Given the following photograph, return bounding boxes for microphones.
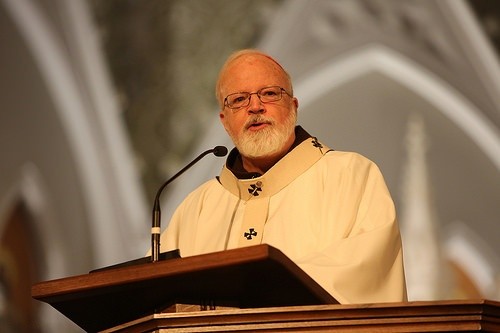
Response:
[152,146,228,262]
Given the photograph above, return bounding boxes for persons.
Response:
[146,50,409,302]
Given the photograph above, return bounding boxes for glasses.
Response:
[223,85,293,109]
